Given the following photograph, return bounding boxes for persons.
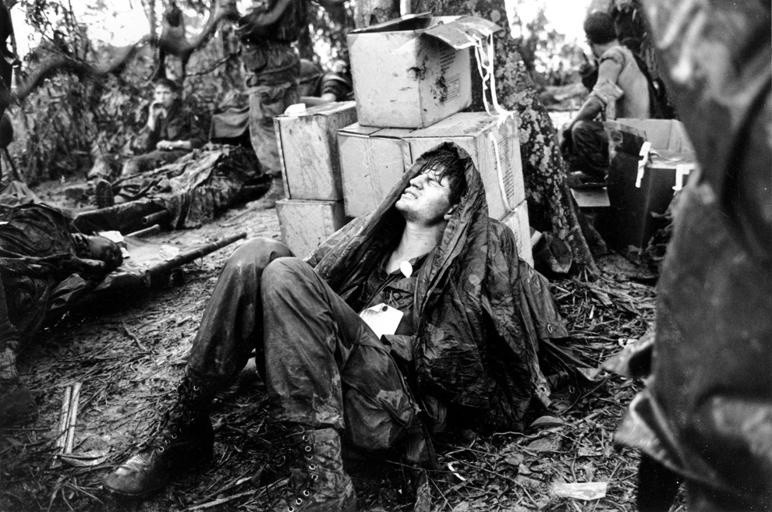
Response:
[89,77,203,211]
[563,12,650,191]
[622,2,771,507]
[297,59,354,106]
[234,0,311,210]
[574,40,599,94]
[101,142,538,512]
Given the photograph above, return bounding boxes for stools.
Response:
[570,186,611,255]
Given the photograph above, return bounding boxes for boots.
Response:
[245,176,286,210]
[275,425,356,511]
[100,367,214,495]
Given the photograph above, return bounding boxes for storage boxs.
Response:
[278,102,358,201]
[408,110,528,222]
[504,200,535,269]
[345,14,503,125]
[278,196,342,261]
[337,124,409,222]
[607,117,697,252]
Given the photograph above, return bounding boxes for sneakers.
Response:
[565,170,607,191]
[62,175,116,209]
